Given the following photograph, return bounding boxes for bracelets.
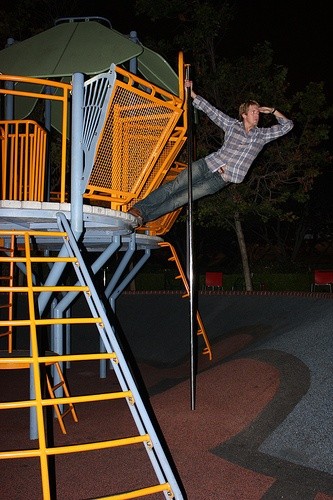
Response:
[271,108,276,114]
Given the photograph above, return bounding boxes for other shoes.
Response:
[129,208,144,226]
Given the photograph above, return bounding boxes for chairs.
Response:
[203,270,224,292]
[309,266,333,293]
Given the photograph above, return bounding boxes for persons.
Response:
[130,80,294,226]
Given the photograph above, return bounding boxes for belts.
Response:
[217,168,224,176]
